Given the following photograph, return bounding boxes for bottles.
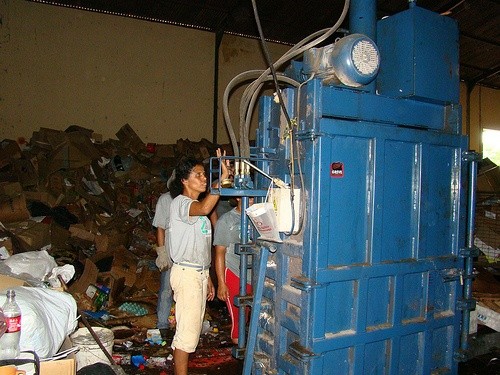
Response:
[0,291,22,358]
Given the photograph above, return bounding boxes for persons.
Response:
[151,165,255,346]
[169,148,230,375]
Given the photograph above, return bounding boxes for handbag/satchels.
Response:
[246,179,282,244]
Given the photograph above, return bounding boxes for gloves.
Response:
[155,246,170,272]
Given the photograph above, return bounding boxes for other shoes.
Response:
[161,329,172,338]
[232,343,240,358]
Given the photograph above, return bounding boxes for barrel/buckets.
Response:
[70,327,114,371]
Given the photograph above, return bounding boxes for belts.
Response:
[175,263,211,272]
